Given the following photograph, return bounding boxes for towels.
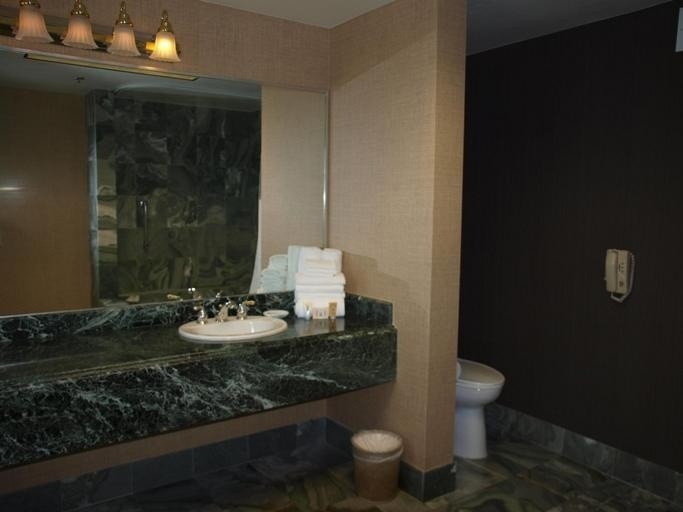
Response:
[256,245,298,295]
[293,247,347,321]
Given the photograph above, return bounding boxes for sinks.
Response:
[177,314,289,344]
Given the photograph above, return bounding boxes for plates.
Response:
[263,309,290,318]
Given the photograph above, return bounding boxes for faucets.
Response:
[188,287,203,298]
[215,299,240,322]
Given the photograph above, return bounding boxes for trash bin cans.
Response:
[350,428,406,501]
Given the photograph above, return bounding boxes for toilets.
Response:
[451,356,505,462]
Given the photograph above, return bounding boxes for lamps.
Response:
[15,0,183,63]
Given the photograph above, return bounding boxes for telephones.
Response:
[604,247,636,303]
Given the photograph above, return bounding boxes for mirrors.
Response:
[0,45,330,320]
